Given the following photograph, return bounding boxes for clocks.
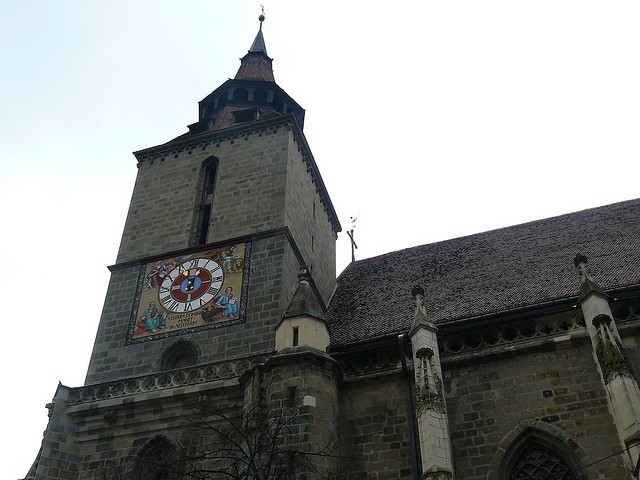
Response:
[157,257,225,314]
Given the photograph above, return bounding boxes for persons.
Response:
[220,245,242,274]
[217,287,238,319]
[141,301,160,332]
[147,260,166,287]
